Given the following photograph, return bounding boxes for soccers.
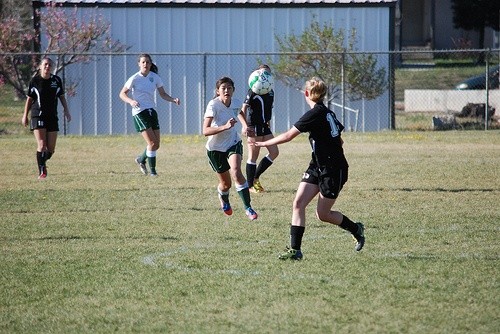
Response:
[247,68,274,95]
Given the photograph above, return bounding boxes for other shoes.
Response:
[37,165,48,177]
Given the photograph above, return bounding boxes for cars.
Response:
[454,65,500,90]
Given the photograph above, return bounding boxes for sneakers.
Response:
[217,196,233,216]
[277,247,302,260]
[250,179,264,195]
[352,222,365,250]
[149,169,158,176]
[244,207,258,219]
[136,155,146,173]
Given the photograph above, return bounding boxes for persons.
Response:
[119,54,181,178]
[201,76,258,221]
[237,63,279,196]
[22,57,73,180]
[247,77,366,262]
